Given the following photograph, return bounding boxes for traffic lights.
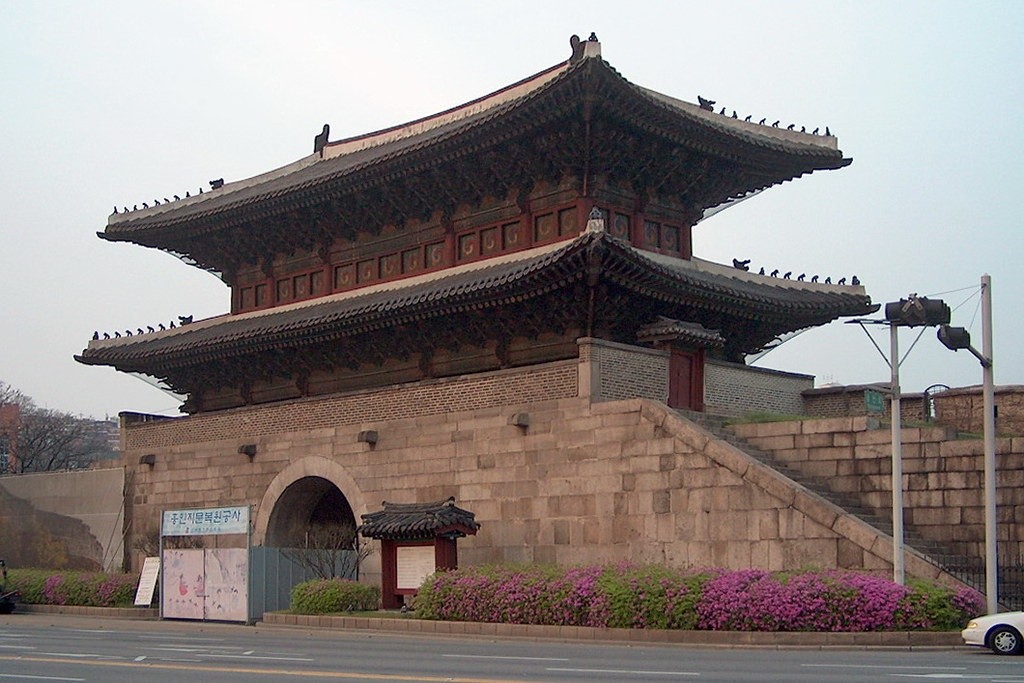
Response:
[917,296,952,328]
[938,327,972,354]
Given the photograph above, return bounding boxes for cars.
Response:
[961,610,1024,655]
